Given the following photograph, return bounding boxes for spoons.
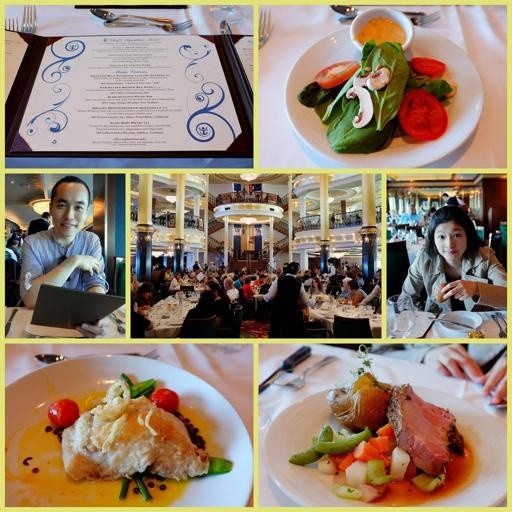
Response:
[111,313,126,335]
[492,314,507,339]
[34,352,68,365]
[89,8,175,23]
[329,4,426,18]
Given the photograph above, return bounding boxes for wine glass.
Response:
[407,234,415,249]
[397,229,407,242]
[422,227,429,243]
[388,294,416,338]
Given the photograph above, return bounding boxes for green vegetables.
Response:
[327,96,398,153]
[426,79,453,96]
[408,77,429,89]
[133,468,164,500]
[297,80,336,107]
[361,39,376,71]
[364,41,410,132]
[118,476,131,500]
[321,65,363,125]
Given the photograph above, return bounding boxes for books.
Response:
[28,283,124,326]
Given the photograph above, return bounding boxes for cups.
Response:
[176,292,183,306]
[255,290,261,296]
[160,302,169,317]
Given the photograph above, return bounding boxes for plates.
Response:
[391,310,437,339]
[4,353,254,508]
[170,319,183,326]
[254,295,266,298]
[262,386,508,509]
[439,310,484,332]
[283,22,485,171]
[25,312,97,339]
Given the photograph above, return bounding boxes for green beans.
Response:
[130,378,156,399]
[313,429,371,455]
[289,425,333,466]
[138,386,154,398]
[187,456,233,477]
[120,373,133,387]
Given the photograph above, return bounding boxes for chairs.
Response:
[215,305,241,338]
[387,240,410,303]
[299,310,333,338]
[187,315,216,338]
[333,315,373,338]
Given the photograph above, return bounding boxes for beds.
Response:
[301,294,382,338]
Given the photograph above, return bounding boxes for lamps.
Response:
[213,202,284,225]
[29,199,51,216]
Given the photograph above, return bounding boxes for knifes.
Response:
[5,308,18,338]
[258,345,312,394]
[428,317,474,331]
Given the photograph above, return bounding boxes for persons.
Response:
[203,282,232,337]
[235,268,273,316]
[179,290,217,339]
[19,177,109,336]
[222,277,239,313]
[182,262,228,293]
[257,263,311,339]
[130,266,186,340]
[401,203,425,230]
[375,342,509,408]
[27,212,50,234]
[303,258,344,296]
[398,207,507,312]
[436,192,473,214]
[425,207,436,223]
[341,260,383,315]
[4,228,29,250]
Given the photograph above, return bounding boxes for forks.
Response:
[257,6,271,48]
[103,19,193,32]
[19,3,38,35]
[338,10,442,26]
[4,16,19,33]
[271,354,338,391]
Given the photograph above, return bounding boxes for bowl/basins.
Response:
[349,6,415,53]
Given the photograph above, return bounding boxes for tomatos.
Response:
[149,388,180,413]
[400,90,448,140]
[315,60,360,89]
[409,55,447,83]
[48,397,79,427]
